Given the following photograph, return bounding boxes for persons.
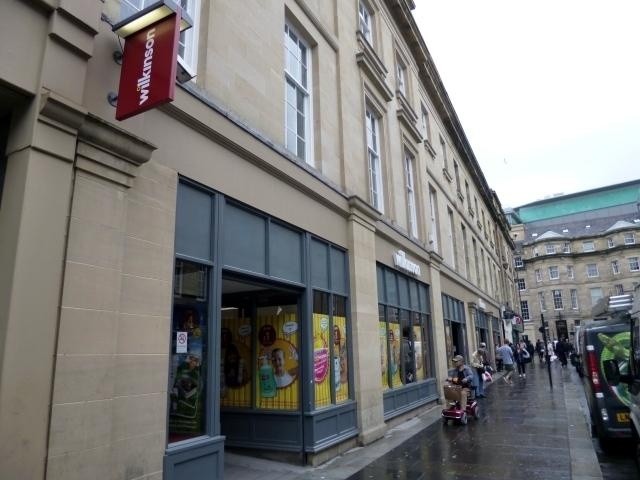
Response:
[271,349,293,389]
[175,355,203,418]
[333,324,343,355]
[513,343,526,379]
[224,343,245,389]
[390,329,397,348]
[451,354,474,413]
[479,343,488,399]
[494,337,570,372]
[496,339,517,386]
[470,347,484,399]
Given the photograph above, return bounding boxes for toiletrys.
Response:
[257,355,277,398]
[334,354,341,392]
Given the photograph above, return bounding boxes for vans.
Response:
[573,282,639,460]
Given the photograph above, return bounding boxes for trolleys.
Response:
[437,375,482,425]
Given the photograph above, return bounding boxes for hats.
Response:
[452,355,464,362]
